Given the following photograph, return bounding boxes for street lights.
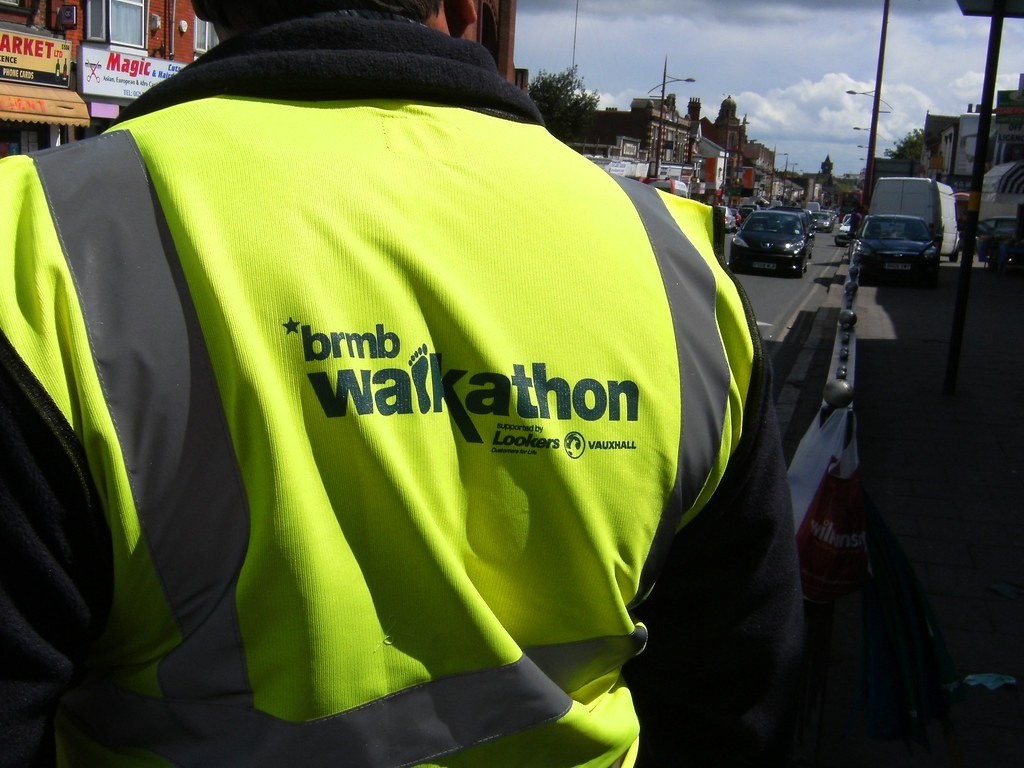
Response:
[846,89,895,207]
[645,54,697,177]
[775,151,805,209]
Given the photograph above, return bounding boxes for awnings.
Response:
[981,160,1024,205]
[0,82,90,128]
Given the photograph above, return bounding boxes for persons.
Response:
[851,208,862,233]
[867,224,883,238]
[0,0,806,768]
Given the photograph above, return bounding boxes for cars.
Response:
[729,207,741,225]
[847,213,944,291]
[725,208,814,278]
[804,199,863,248]
[737,204,759,220]
[717,206,737,234]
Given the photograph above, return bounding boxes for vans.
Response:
[867,176,965,263]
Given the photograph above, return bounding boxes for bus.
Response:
[838,190,864,226]
